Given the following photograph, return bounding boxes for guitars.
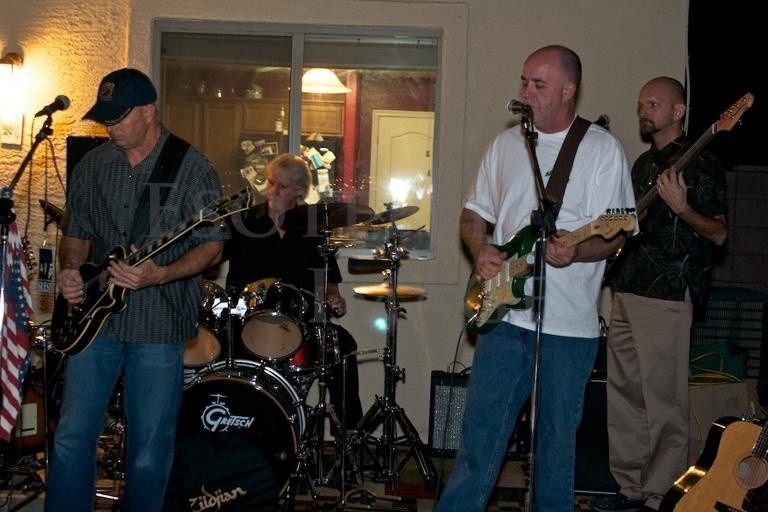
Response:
[604,91,757,274]
[462,208,636,332]
[51,186,258,356]
[658,401,766,511]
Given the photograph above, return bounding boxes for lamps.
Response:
[302,69,352,95]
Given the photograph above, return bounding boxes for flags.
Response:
[0,200,36,444]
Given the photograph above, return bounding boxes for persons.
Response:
[603,76,729,512]
[216,151,375,479]
[433,44,641,512]
[1,187,12,199]
[41,67,227,511]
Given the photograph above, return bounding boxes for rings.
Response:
[657,182,662,188]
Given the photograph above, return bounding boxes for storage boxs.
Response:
[689,378,757,463]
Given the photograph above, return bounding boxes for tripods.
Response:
[302,262,437,511]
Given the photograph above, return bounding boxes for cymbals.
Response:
[38,198,65,228]
[352,283,429,302]
[364,207,420,226]
[278,203,375,231]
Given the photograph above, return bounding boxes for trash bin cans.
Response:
[689,342,750,381]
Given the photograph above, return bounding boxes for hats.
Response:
[79,69,158,126]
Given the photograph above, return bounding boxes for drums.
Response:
[238,280,310,361]
[169,359,307,509]
[183,282,229,366]
[275,321,328,379]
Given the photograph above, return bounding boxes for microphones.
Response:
[506,97,538,116]
[34,96,70,117]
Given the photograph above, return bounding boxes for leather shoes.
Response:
[591,489,640,512]
